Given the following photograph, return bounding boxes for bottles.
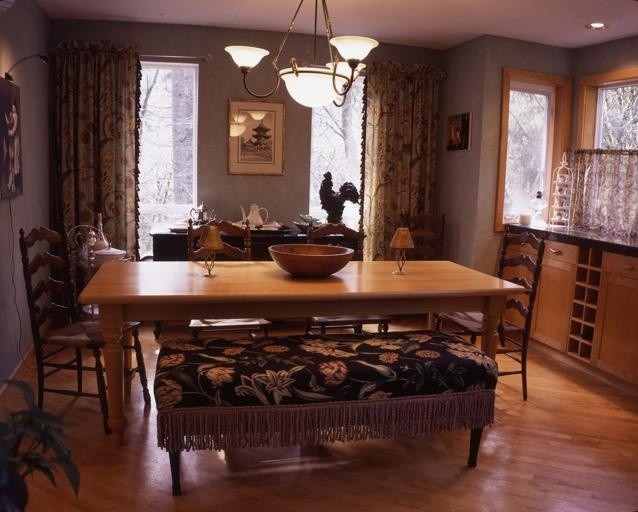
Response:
[94,214,109,250]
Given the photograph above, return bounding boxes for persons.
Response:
[5,101,21,193]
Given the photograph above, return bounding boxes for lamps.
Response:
[222,0,383,112]
[2,54,49,80]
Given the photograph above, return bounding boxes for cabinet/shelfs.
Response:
[506,230,637,387]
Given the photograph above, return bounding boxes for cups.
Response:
[519,208,532,226]
[554,173,572,220]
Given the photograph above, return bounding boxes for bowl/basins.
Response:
[267,243,354,279]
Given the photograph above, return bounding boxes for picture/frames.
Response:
[226,98,286,177]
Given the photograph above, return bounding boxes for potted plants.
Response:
[1,376,83,512]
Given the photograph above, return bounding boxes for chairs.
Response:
[188,218,253,263]
[399,211,547,407]
[17,223,152,438]
[308,219,364,263]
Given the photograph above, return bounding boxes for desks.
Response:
[76,258,527,448]
[150,223,365,260]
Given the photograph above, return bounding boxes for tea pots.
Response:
[190,200,216,225]
[239,202,269,227]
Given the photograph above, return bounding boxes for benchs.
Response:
[151,329,499,497]
